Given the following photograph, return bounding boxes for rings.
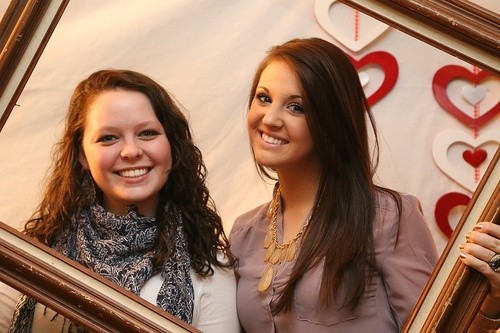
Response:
[490,254,499,272]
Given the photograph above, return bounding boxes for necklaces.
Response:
[258,181,320,292]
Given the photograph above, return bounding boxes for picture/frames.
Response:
[0,0,500,333]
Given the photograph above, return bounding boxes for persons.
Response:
[225,38,500,333]
[18,69,241,333]
